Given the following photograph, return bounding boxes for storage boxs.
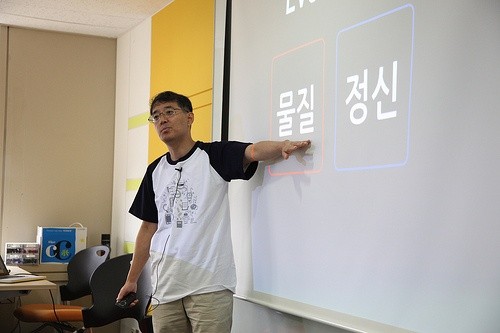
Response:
[37,226,87,264]
[4,242,40,266]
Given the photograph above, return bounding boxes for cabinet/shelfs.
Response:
[0,264,120,333]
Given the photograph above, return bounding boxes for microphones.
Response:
[175,167,183,172]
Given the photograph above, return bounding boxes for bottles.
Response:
[101,234,110,260]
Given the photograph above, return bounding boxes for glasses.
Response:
[148,108,189,122]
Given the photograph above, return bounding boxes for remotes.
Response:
[116,292,136,309]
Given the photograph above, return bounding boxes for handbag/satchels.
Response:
[36,222,87,265]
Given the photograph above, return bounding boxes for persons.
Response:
[116,91,311,333]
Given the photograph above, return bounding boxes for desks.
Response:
[0,266,58,290]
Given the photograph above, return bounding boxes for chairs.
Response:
[13,246,154,333]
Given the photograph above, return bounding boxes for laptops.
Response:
[0,256,47,283]
